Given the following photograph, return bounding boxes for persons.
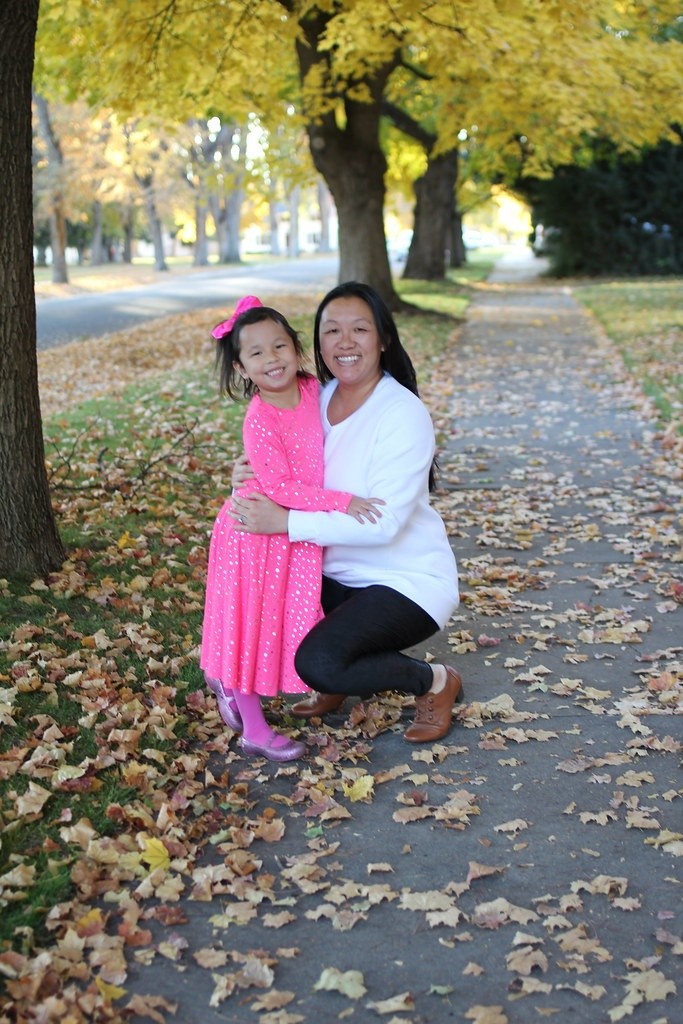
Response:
[227,279,467,744]
[205,302,382,763]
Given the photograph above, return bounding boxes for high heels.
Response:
[291,692,375,717]
[404,663,465,743]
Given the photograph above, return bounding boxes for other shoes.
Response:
[242,733,306,762]
[203,672,243,733]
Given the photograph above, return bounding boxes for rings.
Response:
[239,516,244,524]
